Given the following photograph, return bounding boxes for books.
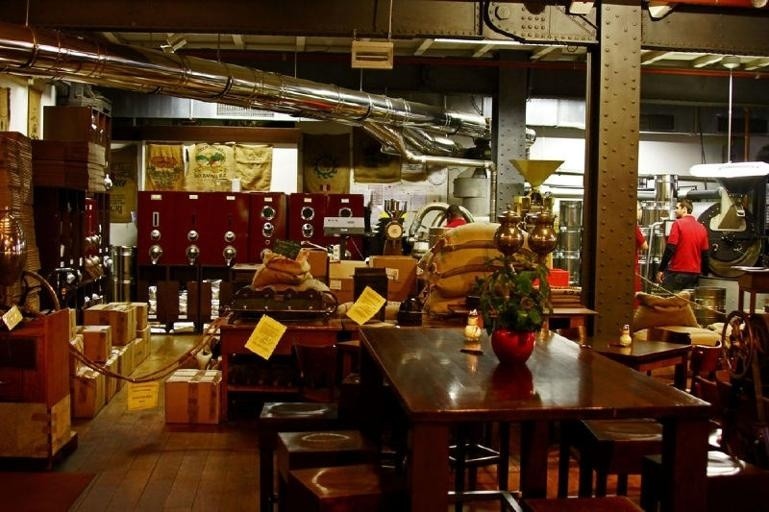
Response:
[163,366,223,428]
[69,298,150,419]
[300,246,419,305]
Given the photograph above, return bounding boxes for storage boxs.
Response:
[300,246,419,305]
[163,366,223,428]
[69,298,150,419]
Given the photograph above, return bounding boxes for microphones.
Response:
[640,449,768,512]
[258,397,401,511]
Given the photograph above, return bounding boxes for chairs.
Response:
[686,339,725,393]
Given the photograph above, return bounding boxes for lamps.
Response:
[686,339,725,393]
[690,67,768,179]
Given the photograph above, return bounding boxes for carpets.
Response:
[1,467,96,511]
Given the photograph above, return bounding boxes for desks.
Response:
[356,326,716,512]
[218,308,344,423]
[737,269,769,316]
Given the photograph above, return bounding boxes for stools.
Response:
[258,397,401,511]
[640,449,768,512]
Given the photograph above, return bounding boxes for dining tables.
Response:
[469,254,553,366]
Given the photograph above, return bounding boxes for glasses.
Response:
[690,67,768,179]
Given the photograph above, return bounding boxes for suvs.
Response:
[696,284,725,325]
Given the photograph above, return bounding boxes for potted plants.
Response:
[469,254,553,366]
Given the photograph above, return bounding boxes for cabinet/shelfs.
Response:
[535,312,552,345]
[60,258,85,286]
[464,313,481,343]
[96,221,111,272]
[618,325,632,348]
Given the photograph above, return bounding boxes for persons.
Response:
[445,205,468,227]
[655,198,710,292]
[633,200,648,311]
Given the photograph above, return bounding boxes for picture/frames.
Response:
[737,269,769,316]
[218,308,344,423]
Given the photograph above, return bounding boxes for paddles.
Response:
[1,467,96,511]
